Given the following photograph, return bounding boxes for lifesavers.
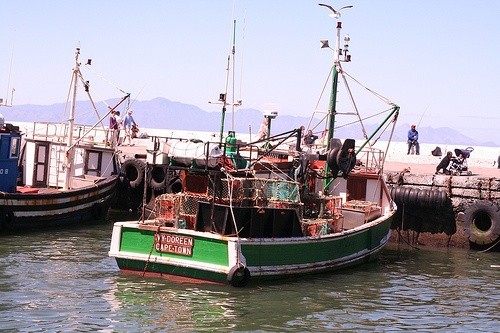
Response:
[145,162,173,192]
[165,175,184,194]
[119,159,146,191]
[461,199,500,245]
[227,265,251,287]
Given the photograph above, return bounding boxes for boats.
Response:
[0,40,120,222]
[108,22,398,288]
[168,56,293,168]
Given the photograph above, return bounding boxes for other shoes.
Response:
[407,152,409,154]
[416,153,419,155]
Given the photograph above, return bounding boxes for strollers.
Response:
[446,147,474,177]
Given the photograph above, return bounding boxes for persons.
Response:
[435,151,452,175]
[110,110,138,147]
[304,130,318,147]
[457,154,463,162]
[407,125,420,155]
[259,118,273,141]
[163,206,187,229]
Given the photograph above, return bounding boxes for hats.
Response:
[411,125,416,128]
[128,109,133,114]
